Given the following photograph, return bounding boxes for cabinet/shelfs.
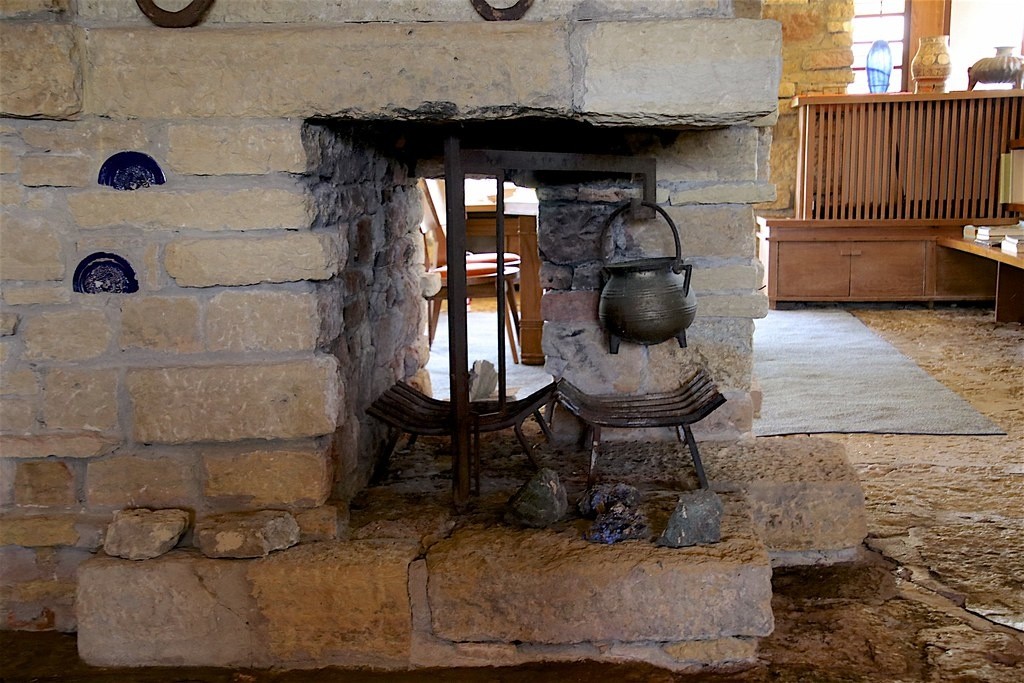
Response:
[755,87,1024,303]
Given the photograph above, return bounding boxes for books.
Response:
[1000,234,1024,255]
[974,223,1024,246]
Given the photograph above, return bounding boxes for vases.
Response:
[910,35,951,92]
[867,39,892,92]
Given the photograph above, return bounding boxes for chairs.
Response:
[417,177,521,364]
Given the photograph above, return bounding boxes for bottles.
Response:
[866,38,893,94]
[911,35,951,94]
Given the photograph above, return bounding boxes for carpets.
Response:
[426,309,1006,435]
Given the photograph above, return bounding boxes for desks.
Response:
[465,193,546,366]
[935,237,1024,323]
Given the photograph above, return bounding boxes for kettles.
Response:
[596,199,697,355]
